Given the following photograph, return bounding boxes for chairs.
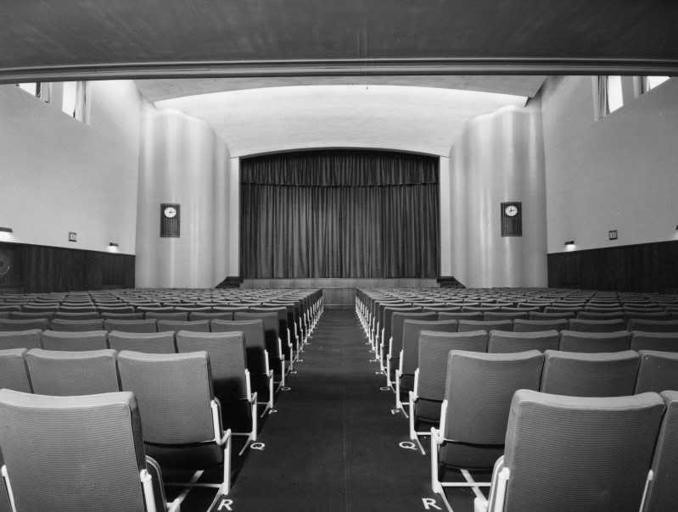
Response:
[407,327,488,443]
[429,348,544,497]
[212,318,277,412]
[1,388,174,512]
[472,388,666,512]
[353,286,678,414]
[176,329,259,456]
[118,349,235,498]
[2,288,325,390]
[25,348,122,398]
[641,388,678,511]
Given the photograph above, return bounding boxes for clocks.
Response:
[160,202,181,237]
[500,201,522,237]
